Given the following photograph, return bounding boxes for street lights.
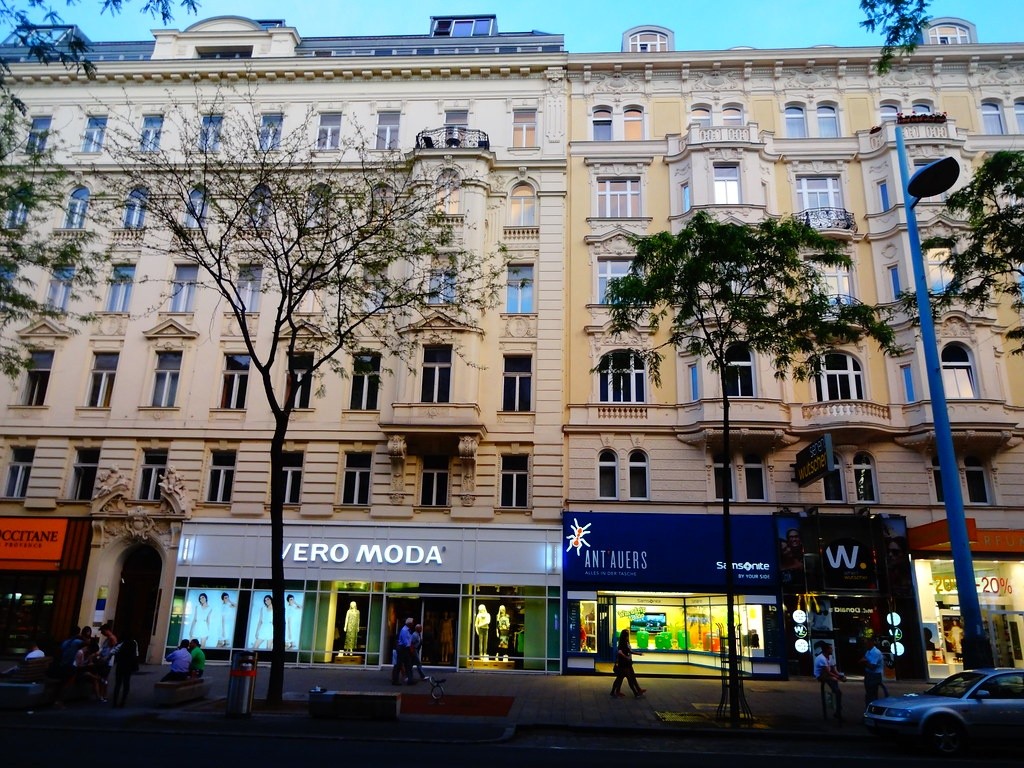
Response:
[894,124,1005,689]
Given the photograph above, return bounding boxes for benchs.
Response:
[310,690,402,719]
[0,683,43,710]
[154,676,214,705]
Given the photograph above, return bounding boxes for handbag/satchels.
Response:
[614,665,621,676]
[66,647,78,664]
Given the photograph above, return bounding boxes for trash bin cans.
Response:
[225,649,258,716]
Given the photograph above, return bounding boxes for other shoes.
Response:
[617,692,625,696]
[392,681,402,686]
[833,713,845,723]
[96,697,108,704]
[639,689,646,695]
[119,703,123,708]
[635,693,639,697]
[112,704,117,708]
[407,679,417,685]
[610,692,615,696]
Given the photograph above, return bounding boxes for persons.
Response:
[858,638,888,701]
[424,612,453,663]
[475,604,491,660]
[814,642,847,721]
[216,592,236,649]
[188,639,205,678]
[951,620,964,663]
[284,594,302,651]
[923,628,935,650]
[889,535,912,586]
[779,528,806,594]
[24,624,140,710]
[160,639,192,683]
[391,618,429,685]
[188,593,212,647]
[610,629,646,699]
[344,601,360,656]
[496,605,510,663]
[253,594,274,650]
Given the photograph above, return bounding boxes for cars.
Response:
[862,666,1024,757]
